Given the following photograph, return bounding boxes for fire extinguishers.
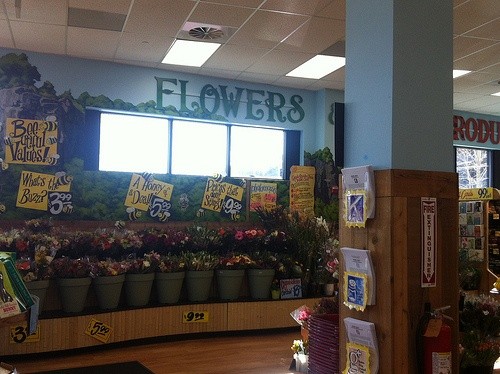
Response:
[423,305,455,374]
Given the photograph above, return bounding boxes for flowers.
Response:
[291,339,308,355]
[459,294,500,374]
[0,205,340,284]
[290,292,339,328]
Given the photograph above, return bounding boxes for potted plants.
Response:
[458,248,482,291]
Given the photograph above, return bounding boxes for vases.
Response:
[324,284,334,295]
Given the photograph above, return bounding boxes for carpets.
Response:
[26,360,154,374]
